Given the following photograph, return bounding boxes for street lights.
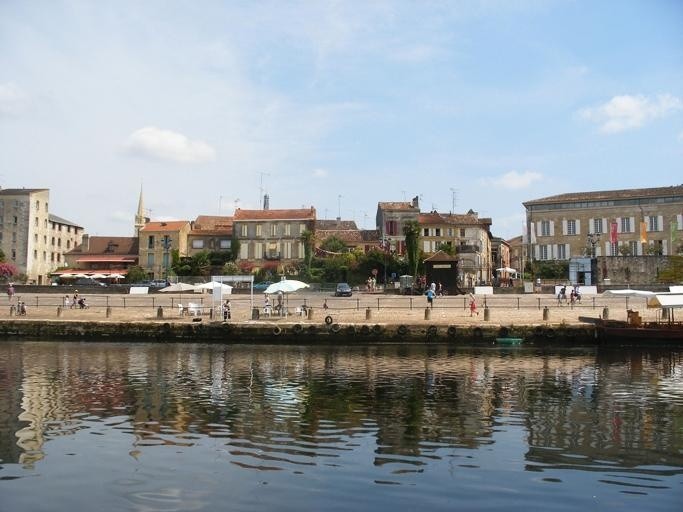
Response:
[380,238,391,284]
[586,232,601,258]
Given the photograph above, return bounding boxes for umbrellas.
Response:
[158,281,196,304]
[264,280,310,305]
[57,272,125,283]
[495,268,517,272]
[196,279,233,308]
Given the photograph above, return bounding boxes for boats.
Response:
[596,309,682,344]
[496,337,525,346]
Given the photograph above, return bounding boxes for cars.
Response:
[253,281,274,290]
[141,279,167,289]
[75,278,101,287]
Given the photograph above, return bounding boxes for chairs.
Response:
[294,307,303,317]
[178,303,204,316]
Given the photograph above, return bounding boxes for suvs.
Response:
[335,283,352,297]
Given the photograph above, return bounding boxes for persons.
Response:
[7,282,14,301]
[21,302,26,316]
[367,275,377,292]
[508,273,513,287]
[224,299,232,320]
[469,293,481,317]
[64,290,87,309]
[17,301,21,313]
[537,277,541,288]
[557,285,582,305]
[425,280,442,308]
[264,292,269,304]
[278,291,283,306]
[323,296,329,310]
